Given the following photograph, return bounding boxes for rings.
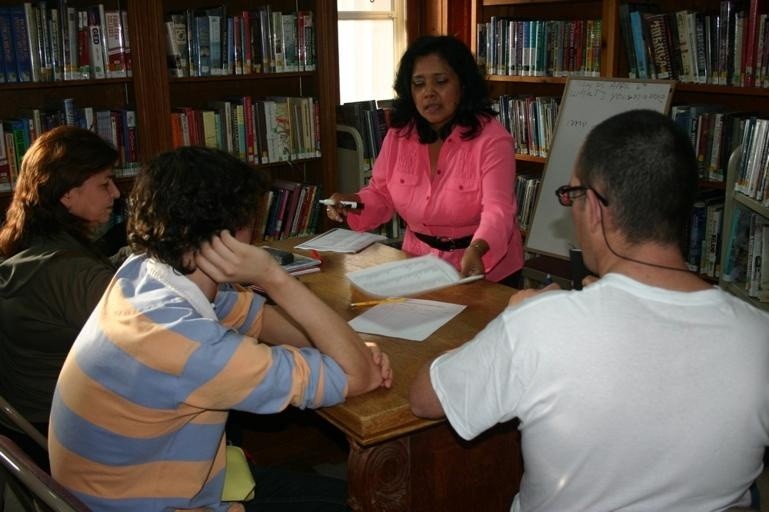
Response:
[326,208,329,212]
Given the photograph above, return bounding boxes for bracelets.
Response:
[471,240,487,254]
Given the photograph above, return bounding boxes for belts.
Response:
[409,227,476,254]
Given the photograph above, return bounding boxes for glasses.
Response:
[553,179,612,210]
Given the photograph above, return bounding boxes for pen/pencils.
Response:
[350,298,408,306]
[319,199,361,209]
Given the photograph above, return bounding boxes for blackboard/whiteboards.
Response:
[523,75,677,263]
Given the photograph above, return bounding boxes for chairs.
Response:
[0,434,91,512]
[0,395,48,453]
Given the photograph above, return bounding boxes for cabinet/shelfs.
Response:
[0,0,343,243]
[717,144,769,315]
[472,0,769,285]
[335,125,406,249]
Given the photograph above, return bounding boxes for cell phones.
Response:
[259,244,295,265]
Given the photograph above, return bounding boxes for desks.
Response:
[243,235,523,512]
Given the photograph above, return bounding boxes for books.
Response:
[669,105,768,302]
[250,184,323,241]
[163,2,316,79]
[1,2,133,86]
[1,96,137,185]
[242,244,322,293]
[489,93,558,233]
[628,1,768,88]
[474,15,604,77]
[169,92,322,167]
[336,98,409,241]
[294,226,388,254]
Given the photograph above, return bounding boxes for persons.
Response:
[404,104,768,510]
[325,33,526,291]
[42,142,397,512]
[1,120,130,480]
